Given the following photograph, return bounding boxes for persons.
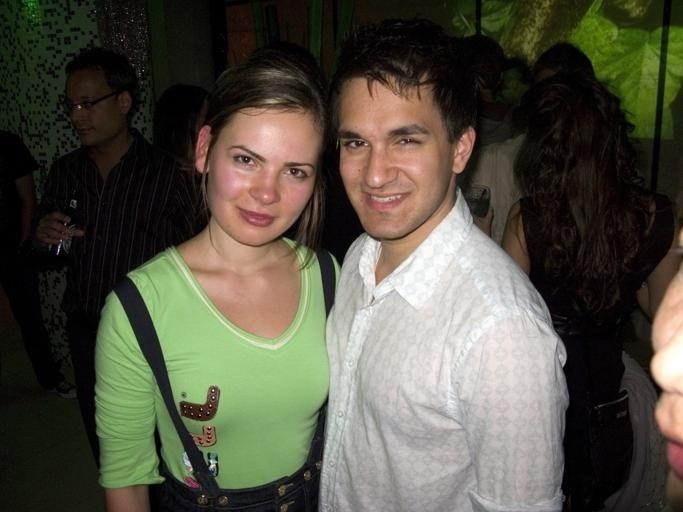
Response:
[94,55,342,510]
[2,48,211,509]
[461,42,683,510]
[317,31,569,511]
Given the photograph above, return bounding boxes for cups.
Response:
[466,183,491,226]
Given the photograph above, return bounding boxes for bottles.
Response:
[50,186,81,260]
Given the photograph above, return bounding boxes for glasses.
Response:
[61,93,114,114]
